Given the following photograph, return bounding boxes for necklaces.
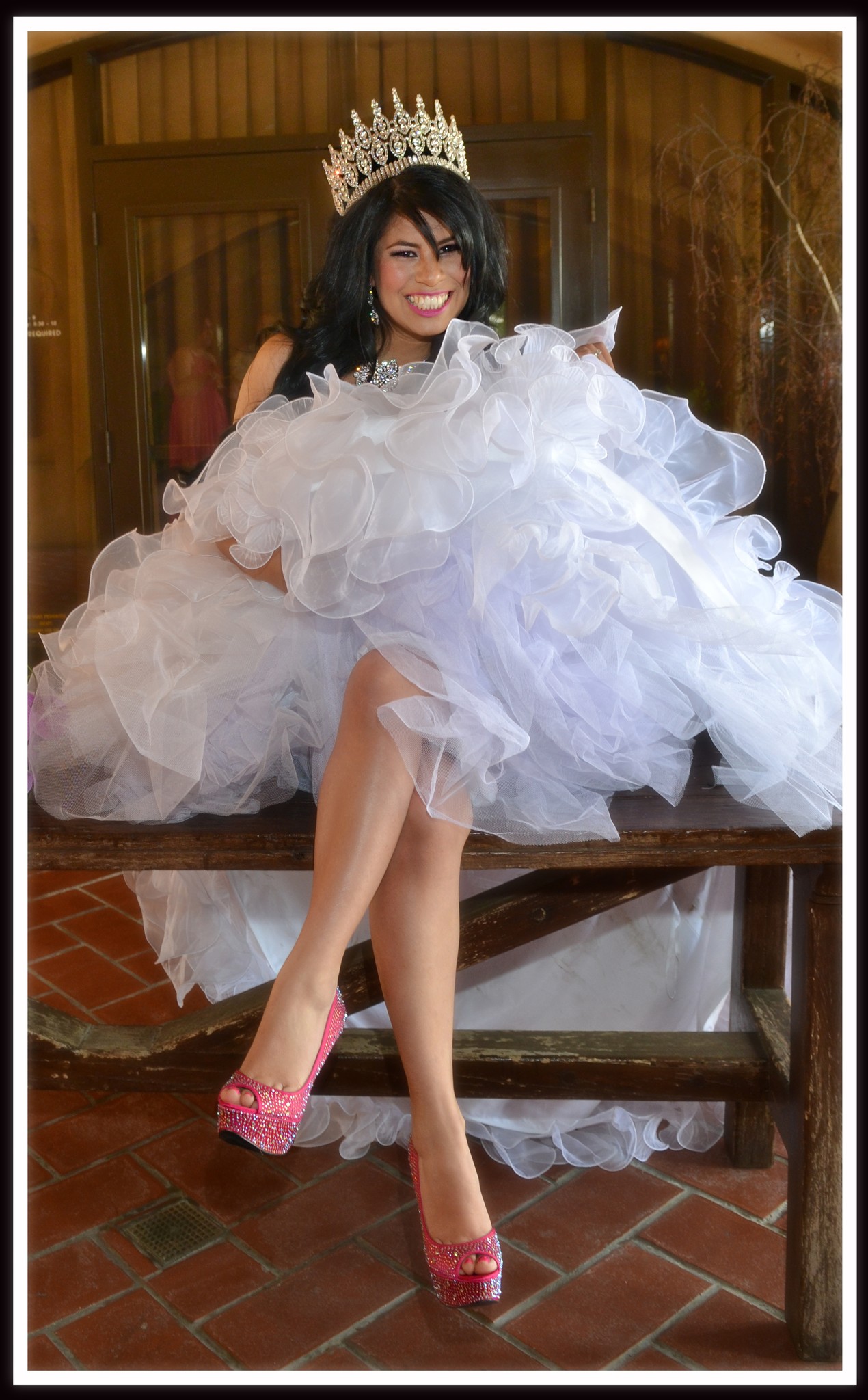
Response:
[354,358,429,392]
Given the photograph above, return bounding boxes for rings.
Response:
[595,348,602,357]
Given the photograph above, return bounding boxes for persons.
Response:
[30,85,840,1306]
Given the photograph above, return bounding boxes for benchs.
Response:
[27,730,842,1363]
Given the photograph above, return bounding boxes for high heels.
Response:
[409,1134,503,1306]
[217,985,347,1158]
